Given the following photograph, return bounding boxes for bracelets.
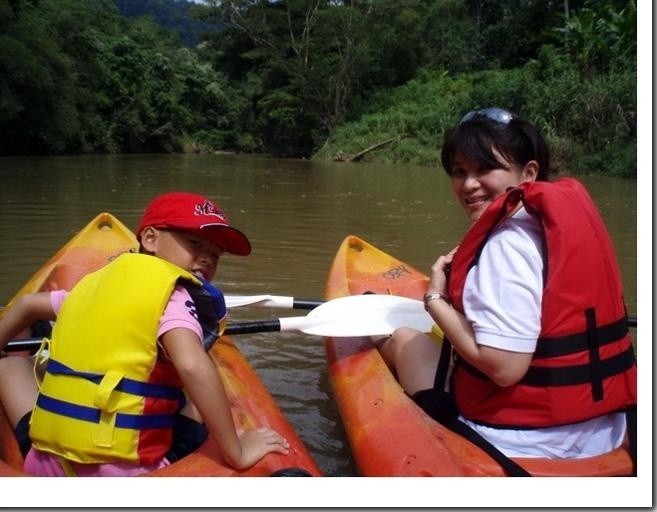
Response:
[423,291,451,310]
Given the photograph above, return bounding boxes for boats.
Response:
[318,233,634,477]
[0,208,323,477]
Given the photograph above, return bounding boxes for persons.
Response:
[359,104,636,460]
[0,189,290,477]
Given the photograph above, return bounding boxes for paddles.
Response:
[0,293,435,350]
[224,295,636,327]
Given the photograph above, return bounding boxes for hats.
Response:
[136,192,251,255]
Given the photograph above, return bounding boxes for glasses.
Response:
[458,107,512,125]
